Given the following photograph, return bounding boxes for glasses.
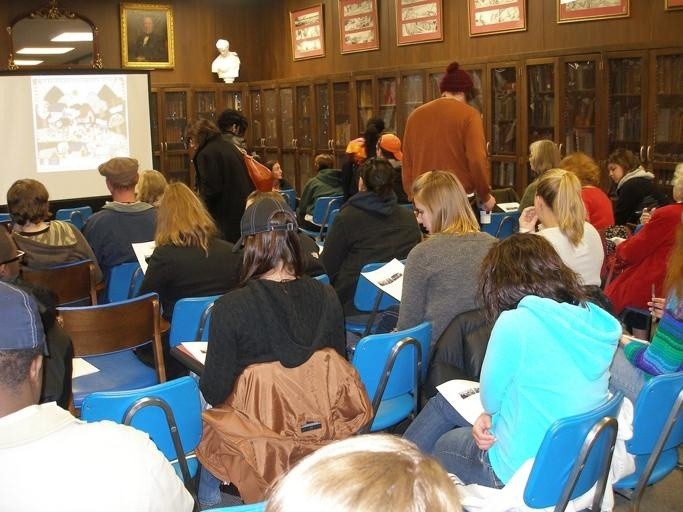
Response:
[0,249,27,265]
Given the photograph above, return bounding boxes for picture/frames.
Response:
[120,1,176,71]
[288,3,325,62]
[466,0,527,38]
[337,0,379,55]
[664,0,683,12]
[394,0,444,46]
[556,0,630,24]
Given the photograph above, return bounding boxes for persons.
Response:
[134,16,165,62]
[0,55,682,512]
[211,39,240,77]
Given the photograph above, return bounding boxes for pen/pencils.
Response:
[651,284,656,322]
[634,211,652,214]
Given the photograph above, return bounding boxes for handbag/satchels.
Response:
[605,223,633,275]
[228,137,273,192]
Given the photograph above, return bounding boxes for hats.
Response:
[231,194,300,255]
[377,132,403,162]
[438,60,474,95]
[0,280,53,364]
[98,156,139,184]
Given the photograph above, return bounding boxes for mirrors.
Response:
[4,0,103,71]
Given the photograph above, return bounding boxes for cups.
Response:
[479,210,492,225]
